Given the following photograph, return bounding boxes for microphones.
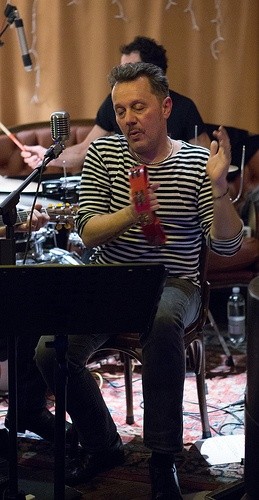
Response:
[14,9,32,72]
[44,112,70,158]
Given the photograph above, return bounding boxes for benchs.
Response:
[0,116,259,293]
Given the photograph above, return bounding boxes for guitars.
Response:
[0,202,80,231]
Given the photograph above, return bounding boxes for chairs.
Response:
[103,235,211,442]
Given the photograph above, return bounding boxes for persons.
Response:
[21,37,211,170]
[34,62,242,500]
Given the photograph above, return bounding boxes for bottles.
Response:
[227,287,246,345]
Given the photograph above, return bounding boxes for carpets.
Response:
[0,347,242,492]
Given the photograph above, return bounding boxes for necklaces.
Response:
[134,136,173,165]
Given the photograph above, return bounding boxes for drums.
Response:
[0,206,44,258]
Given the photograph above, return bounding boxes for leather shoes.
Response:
[65,432,125,486]
[149,457,183,500]
[4,408,73,444]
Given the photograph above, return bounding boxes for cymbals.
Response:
[128,163,165,247]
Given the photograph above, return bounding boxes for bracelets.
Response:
[212,187,229,200]
